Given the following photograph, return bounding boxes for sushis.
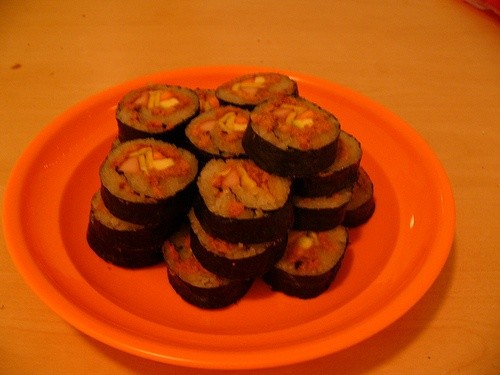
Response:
[86,72,375,308]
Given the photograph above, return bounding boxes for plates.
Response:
[0,64,468,373]
